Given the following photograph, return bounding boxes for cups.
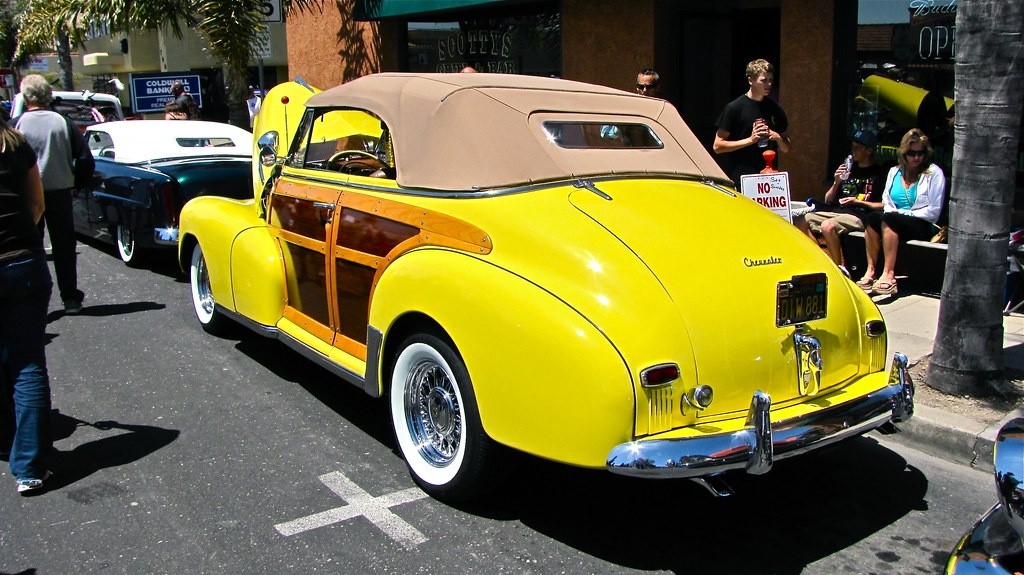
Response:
[756,119,769,147]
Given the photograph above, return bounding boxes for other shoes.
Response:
[64,300,83,314]
[16,477,43,492]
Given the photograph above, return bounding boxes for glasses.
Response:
[636,84,655,90]
[907,149,925,157]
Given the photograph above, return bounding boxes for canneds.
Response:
[755,118,768,147]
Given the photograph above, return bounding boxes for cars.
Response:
[73,119,254,268]
[177,70,917,508]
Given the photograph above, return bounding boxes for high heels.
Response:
[856,276,897,294]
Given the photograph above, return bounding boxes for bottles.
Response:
[839,155,852,180]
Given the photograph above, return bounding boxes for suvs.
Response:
[9,90,124,136]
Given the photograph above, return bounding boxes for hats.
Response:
[249,85,254,92]
[850,129,878,148]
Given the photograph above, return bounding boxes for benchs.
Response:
[808,220,949,252]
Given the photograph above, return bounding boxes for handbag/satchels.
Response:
[930,224,948,243]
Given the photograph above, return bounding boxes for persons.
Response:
[713,59,792,192]
[460,66,477,74]
[620,68,661,147]
[0,98,62,494]
[244,85,262,132]
[7,74,85,313]
[165,81,200,121]
[804,128,945,294]
